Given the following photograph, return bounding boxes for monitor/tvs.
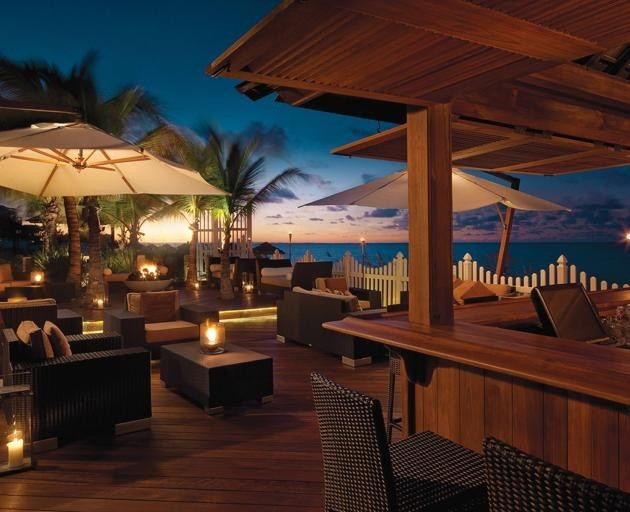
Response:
[530,281,610,345]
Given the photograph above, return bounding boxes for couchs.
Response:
[0,258,39,299]
[254,257,334,302]
[283,284,384,367]
[102,285,221,361]
[206,255,253,293]
[3,322,153,451]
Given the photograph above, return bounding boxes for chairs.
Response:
[480,436,629,511]
[311,372,486,511]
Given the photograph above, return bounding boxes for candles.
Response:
[6,438,24,468]
[6,429,24,443]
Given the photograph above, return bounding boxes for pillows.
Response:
[326,277,346,294]
[315,277,331,294]
[17,321,47,361]
[127,291,181,323]
[44,318,68,358]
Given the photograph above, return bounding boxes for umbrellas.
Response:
[297,167,572,279]
[252,242,285,259]
[0,106,232,197]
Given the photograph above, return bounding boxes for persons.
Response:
[272,249,282,260]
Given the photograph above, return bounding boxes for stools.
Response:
[380,302,411,439]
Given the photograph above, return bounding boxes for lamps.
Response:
[198,320,225,355]
[29,270,44,282]
